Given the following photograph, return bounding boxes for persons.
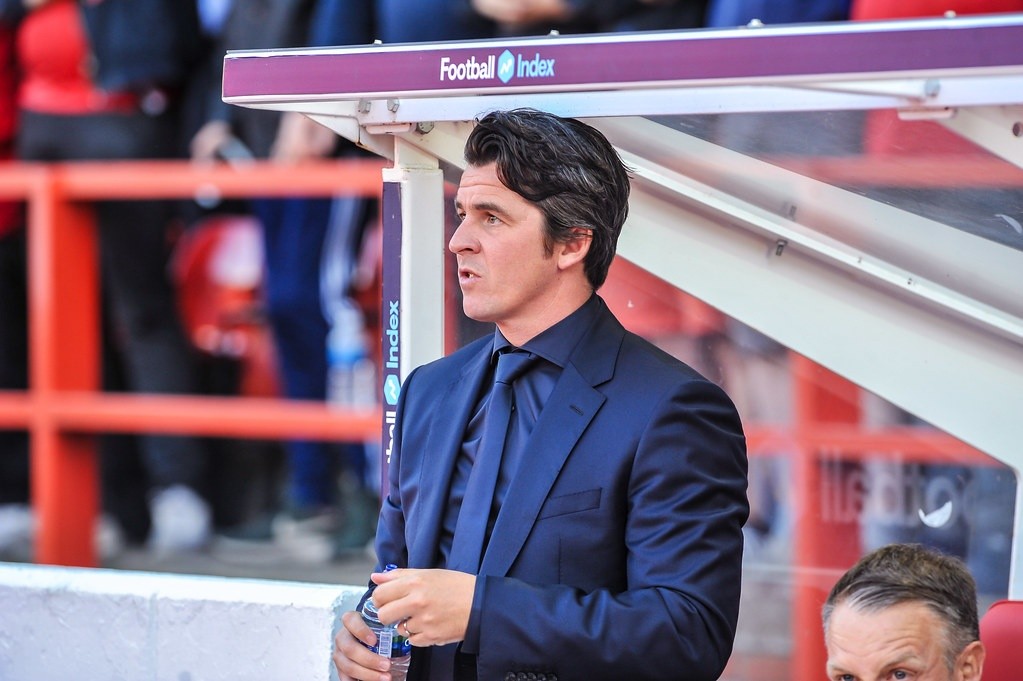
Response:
[0,0,1023,594]
[331,108,749,681]
[184,0,1023,602]
[822,543,986,681]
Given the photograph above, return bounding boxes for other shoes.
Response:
[0,499,124,563]
[270,509,344,565]
[143,485,215,555]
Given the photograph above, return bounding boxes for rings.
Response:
[402,619,412,636]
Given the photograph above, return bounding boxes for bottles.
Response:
[361,564,411,681]
[325,309,371,411]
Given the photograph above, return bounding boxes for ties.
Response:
[420,350,540,681]
